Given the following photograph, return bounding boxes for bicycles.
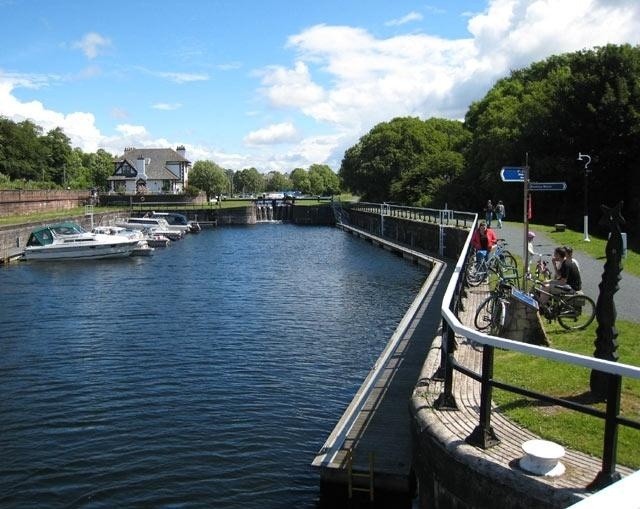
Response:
[465,238,595,330]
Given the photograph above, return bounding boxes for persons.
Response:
[536,247,582,310]
[471,221,498,285]
[550,245,581,274]
[483,199,494,230]
[527,231,536,267]
[494,200,506,229]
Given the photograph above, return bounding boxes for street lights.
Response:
[444,175,451,215]
[577,152,591,242]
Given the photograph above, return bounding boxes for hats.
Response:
[528,231,535,237]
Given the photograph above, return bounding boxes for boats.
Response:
[17,211,201,261]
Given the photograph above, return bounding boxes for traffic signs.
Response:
[528,182,567,191]
[500,167,525,182]
